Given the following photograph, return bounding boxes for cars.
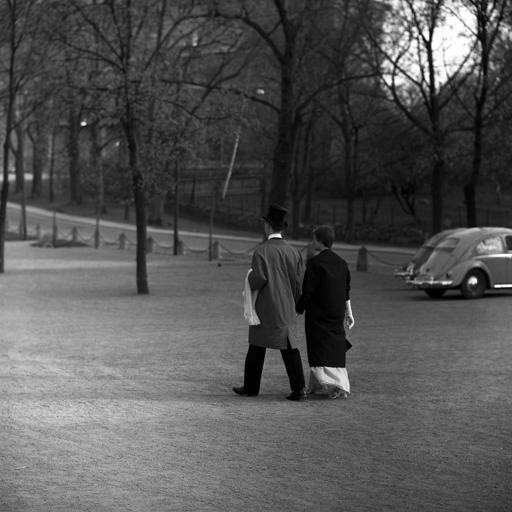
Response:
[392,226,512,300]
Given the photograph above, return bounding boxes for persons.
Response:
[295,224,355,397]
[231,218,308,402]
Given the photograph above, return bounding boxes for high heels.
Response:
[305,383,348,399]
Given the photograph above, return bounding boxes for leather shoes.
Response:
[232,386,259,397]
[286,390,308,400]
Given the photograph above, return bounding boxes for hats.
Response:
[260,204,289,230]
